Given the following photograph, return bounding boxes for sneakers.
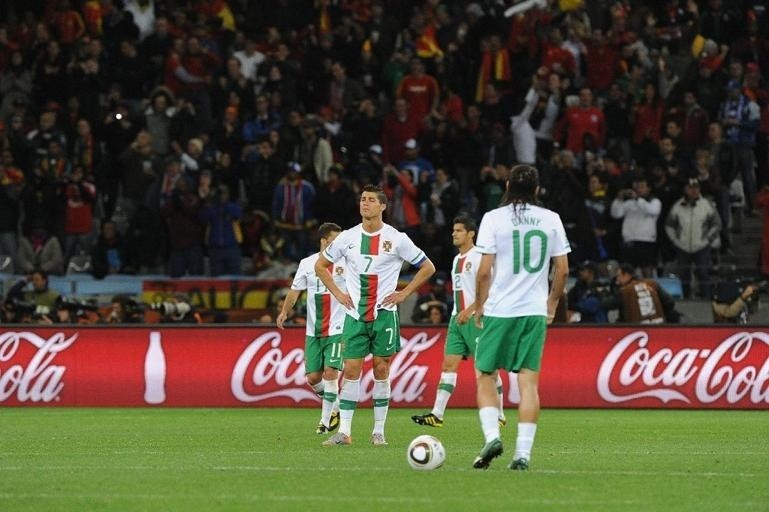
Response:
[316,424,328,435]
[370,432,384,446]
[498,417,507,429]
[507,458,529,470]
[472,438,503,469]
[329,411,340,431]
[412,412,444,428]
[321,431,352,447]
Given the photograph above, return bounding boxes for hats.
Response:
[404,138,420,150]
[576,259,598,271]
[369,144,384,154]
[287,160,301,172]
[148,85,177,106]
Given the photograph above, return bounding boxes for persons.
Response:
[314,182,436,449]
[472,163,573,471]
[1,1,768,322]
[277,222,347,436]
[411,214,507,430]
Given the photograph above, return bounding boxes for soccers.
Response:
[406,435,446,468]
